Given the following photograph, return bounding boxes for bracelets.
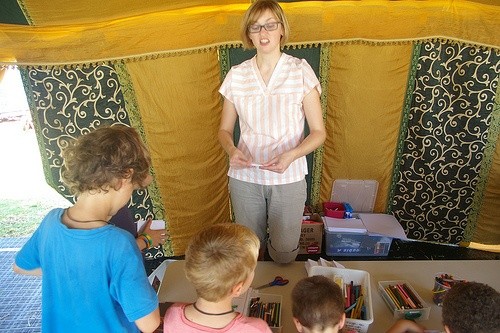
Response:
[139,232,153,248]
[138,235,150,248]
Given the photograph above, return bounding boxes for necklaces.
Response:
[192,301,235,315]
[66,206,111,225]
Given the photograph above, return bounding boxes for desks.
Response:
[148,260,500,333]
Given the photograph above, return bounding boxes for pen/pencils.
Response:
[335,208,338,211]
[232,305,238,308]
[330,209,332,210]
[384,283,424,310]
[335,276,366,320]
[326,208,329,210]
[436,273,466,287]
[251,163,275,167]
[249,296,280,327]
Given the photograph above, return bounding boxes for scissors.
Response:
[254,276,289,289]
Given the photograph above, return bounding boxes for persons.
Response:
[13,125,169,333]
[163,223,274,333]
[386,282,500,333]
[217,0,327,264]
[292,276,358,333]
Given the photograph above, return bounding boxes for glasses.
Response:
[247,20,283,33]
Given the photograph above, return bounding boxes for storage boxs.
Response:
[296,214,323,253]
[321,213,393,256]
